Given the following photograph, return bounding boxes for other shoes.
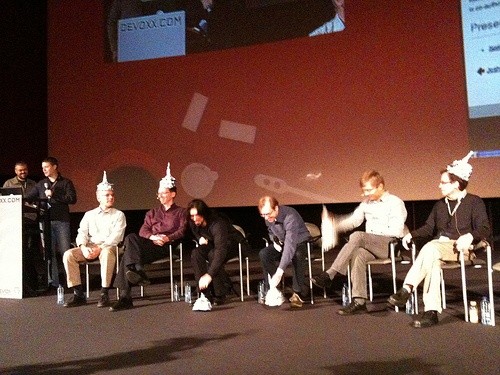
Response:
[43,284,60,297]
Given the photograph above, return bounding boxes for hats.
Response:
[97,171,114,191]
[159,162,176,188]
[447,151,473,181]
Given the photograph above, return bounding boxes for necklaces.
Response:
[445,190,467,216]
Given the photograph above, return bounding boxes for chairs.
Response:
[70,223,495,324]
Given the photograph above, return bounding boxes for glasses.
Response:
[260,210,274,217]
[441,181,452,185]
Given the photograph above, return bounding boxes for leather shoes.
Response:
[109,298,133,311]
[63,295,85,307]
[337,301,368,315]
[213,297,226,306]
[97,295,109,307]
[390,288,410,307]
[311,272,332,289]
[126,269,151,287]
[413,312,437,328]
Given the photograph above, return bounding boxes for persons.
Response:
[109,0,246,62]
[187,199,246,305]
[107,181,188,311]
[384,169,490,327]
[3,160,46,292]
[63,182,126,307]
[309,0,346,37]
[22,157,76,294]
[257,195,313,308]
[310,169,408,315]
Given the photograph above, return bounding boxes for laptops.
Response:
[0,187,38,211]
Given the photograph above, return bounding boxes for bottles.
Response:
[469,301,478,323]
[57,283,64,304]
[405,292,415,314]
[184,282,192,302]
[480,296,491,326]
[257,279,266,305]
[342,283,349,307]
[173,280,181,301]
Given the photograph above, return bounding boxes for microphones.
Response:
[44,182,51,200]
